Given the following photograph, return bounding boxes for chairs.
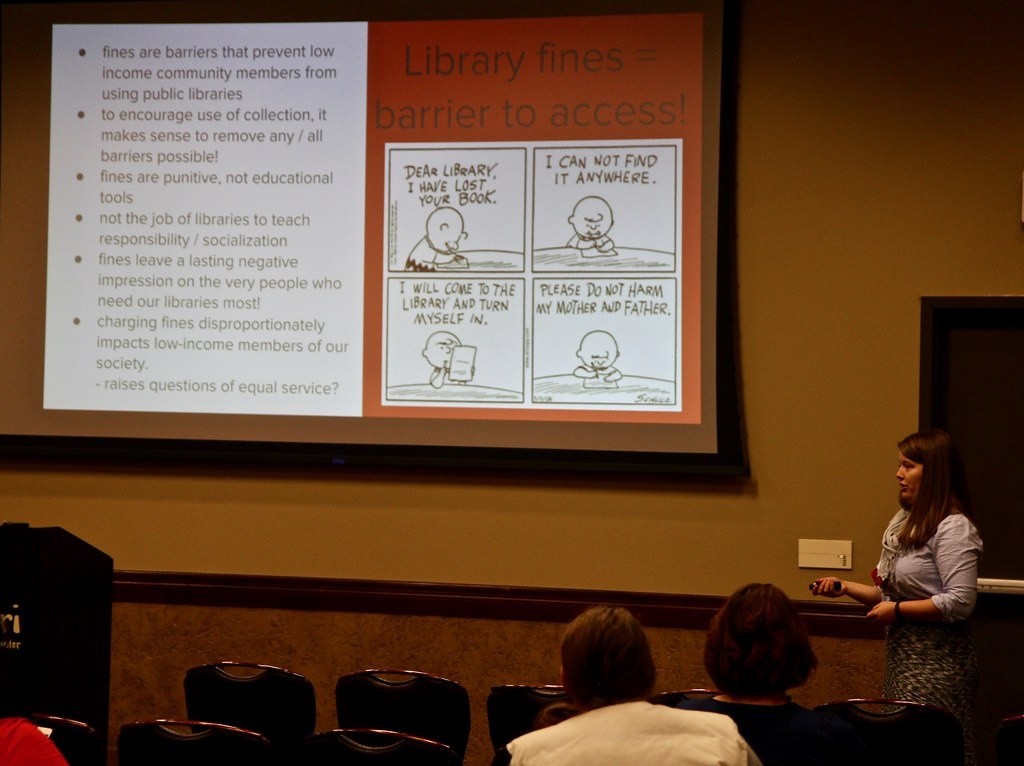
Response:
[31,662,1024,766]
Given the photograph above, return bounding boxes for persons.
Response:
[491,607,763,766]
[812,429,983,766]
[675,583,876,766]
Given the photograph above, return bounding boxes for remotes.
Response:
[808,581,842,591]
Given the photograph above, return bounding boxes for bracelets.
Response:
[894,601,904,621]
